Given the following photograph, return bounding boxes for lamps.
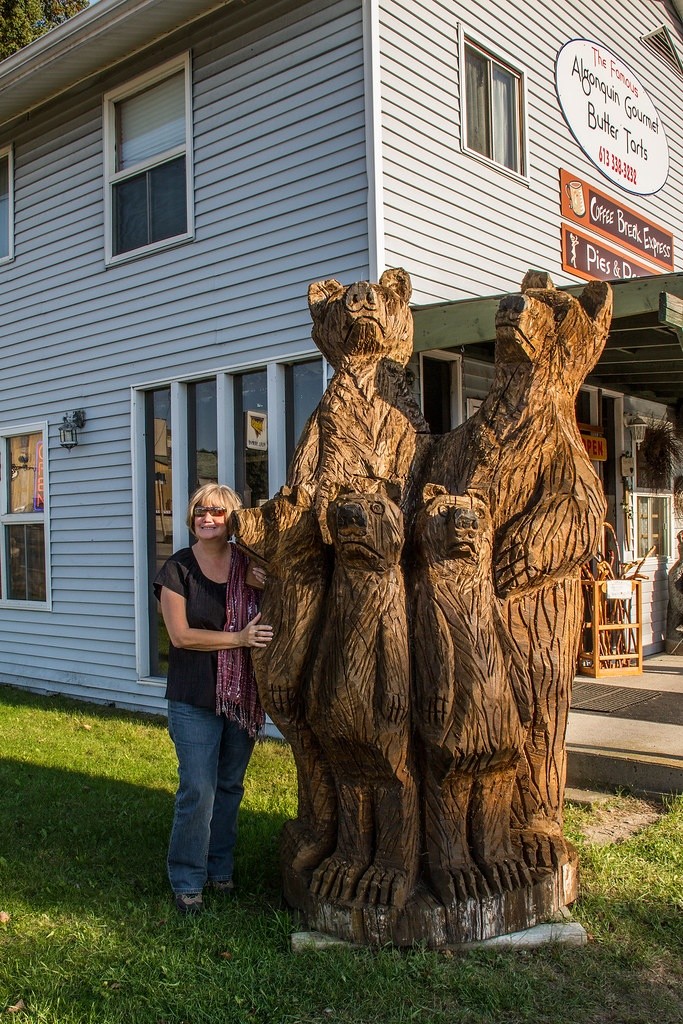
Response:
[58,410,86,454]
[624,410,646,449]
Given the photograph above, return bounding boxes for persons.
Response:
[153,483,274,914]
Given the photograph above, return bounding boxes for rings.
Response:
[262,581,265,584]
[263,576,268,582]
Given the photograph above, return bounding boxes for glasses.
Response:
[194,506,227,517]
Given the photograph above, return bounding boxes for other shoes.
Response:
[176,893,203,912]
[213,880,234,897]
